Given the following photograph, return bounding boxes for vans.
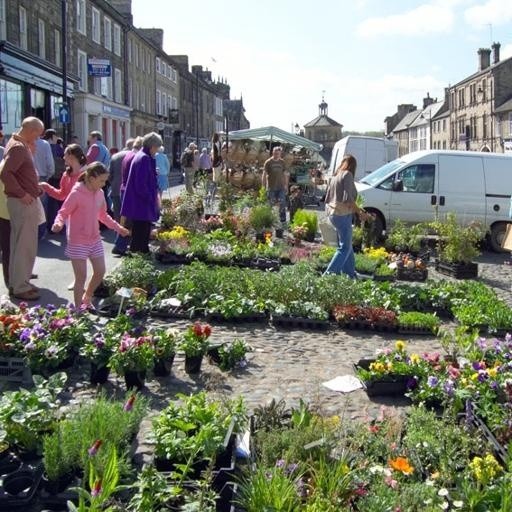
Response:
[324,149,511,253]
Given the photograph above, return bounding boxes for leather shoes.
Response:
[10,284,40,299]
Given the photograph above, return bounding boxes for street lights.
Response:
[477,80,492,152]
[418,109,432,150]
[196,70,212,151]
[295,123,300,135]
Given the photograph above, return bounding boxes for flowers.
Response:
[0,188,511,511]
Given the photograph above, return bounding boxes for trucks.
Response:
[385,142,399,179]
[328,135,386,184]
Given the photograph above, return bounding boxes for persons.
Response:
[261,147,289,222]
[0,116,171,312]
[178,141,212,195]
[320,154,368,278]
